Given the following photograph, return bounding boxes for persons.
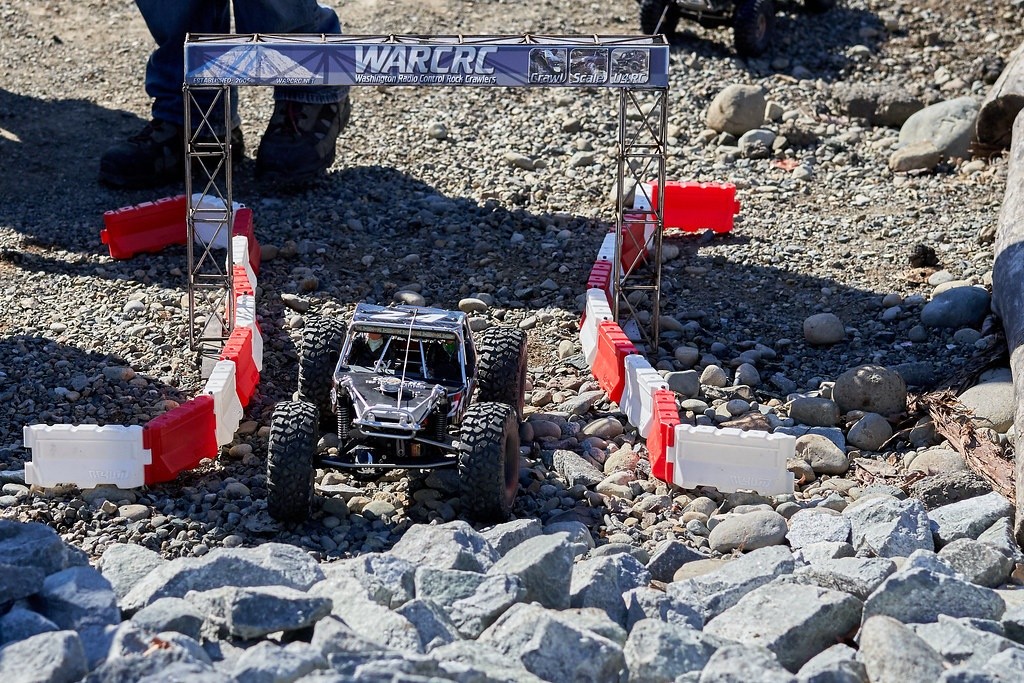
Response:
[97,0,352,193]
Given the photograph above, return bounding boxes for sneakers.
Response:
[98,116,243,186]
[252,95,349,186]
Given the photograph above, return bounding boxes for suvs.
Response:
[267,299,529,528]
[638,0,838,61]
